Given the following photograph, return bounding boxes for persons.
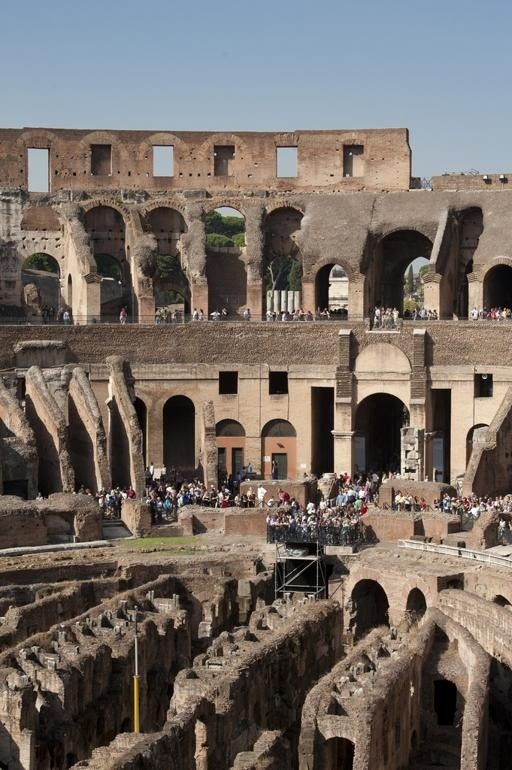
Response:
[394,490,512,518]
[35,492,43,501]
[71,485,136,520]
[499,518,506,530]
[144,463,268,523]
[265,307,330,321]
[382,471,401,483]
[266,470,379,543]
[119,306,127,322]
[156,307,227,324]
[374,306,438,321]
[470,307,512,321]
[243,308,251,321]
[41,304,71,325]
